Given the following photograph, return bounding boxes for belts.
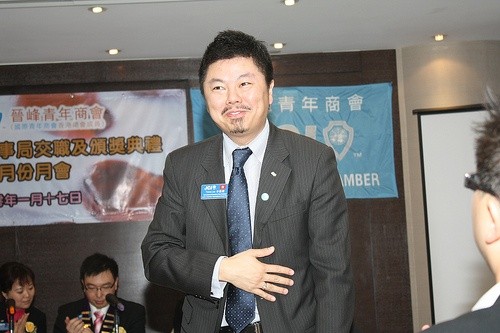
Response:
[220,321,262,333]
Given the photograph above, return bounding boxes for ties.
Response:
[225,147,256,333]
[93,312,106,325]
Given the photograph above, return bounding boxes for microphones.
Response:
[105,294,124,311]
[7,298,16,333]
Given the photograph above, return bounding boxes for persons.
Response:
[52,252,146,333]
[412,93,500,333]
[0,261,47,333]
[140,29,356,333]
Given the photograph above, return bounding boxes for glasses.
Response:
[466,173,500,200]
[83,279,116,293]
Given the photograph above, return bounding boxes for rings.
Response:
[262,283,267,290]
